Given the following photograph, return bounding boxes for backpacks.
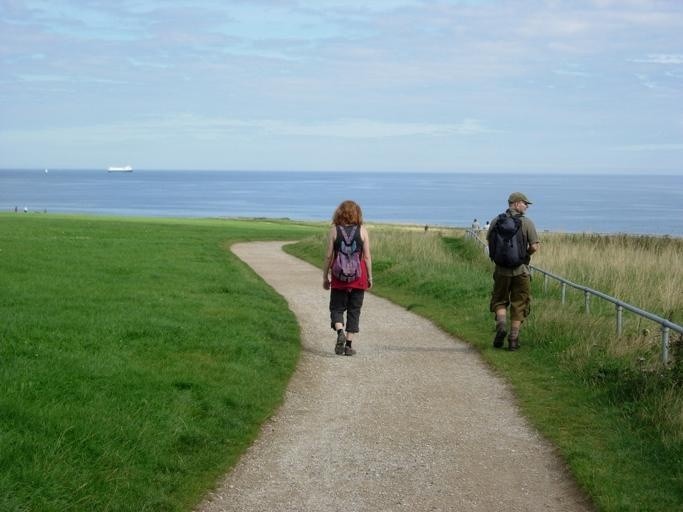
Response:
[489,213,526,267]
[332,224,362,282]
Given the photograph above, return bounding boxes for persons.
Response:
[471,218,480,238]
[483,220,490,231]
[322,199,373,356]
[484,191,540,351]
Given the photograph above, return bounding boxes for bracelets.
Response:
[367,278,372,281]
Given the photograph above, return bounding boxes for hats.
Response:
[508,192,532,204]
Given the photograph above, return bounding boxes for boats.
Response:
[107,165,133,172]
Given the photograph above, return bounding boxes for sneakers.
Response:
[494,328,520,350]
[335,334,352,356]
